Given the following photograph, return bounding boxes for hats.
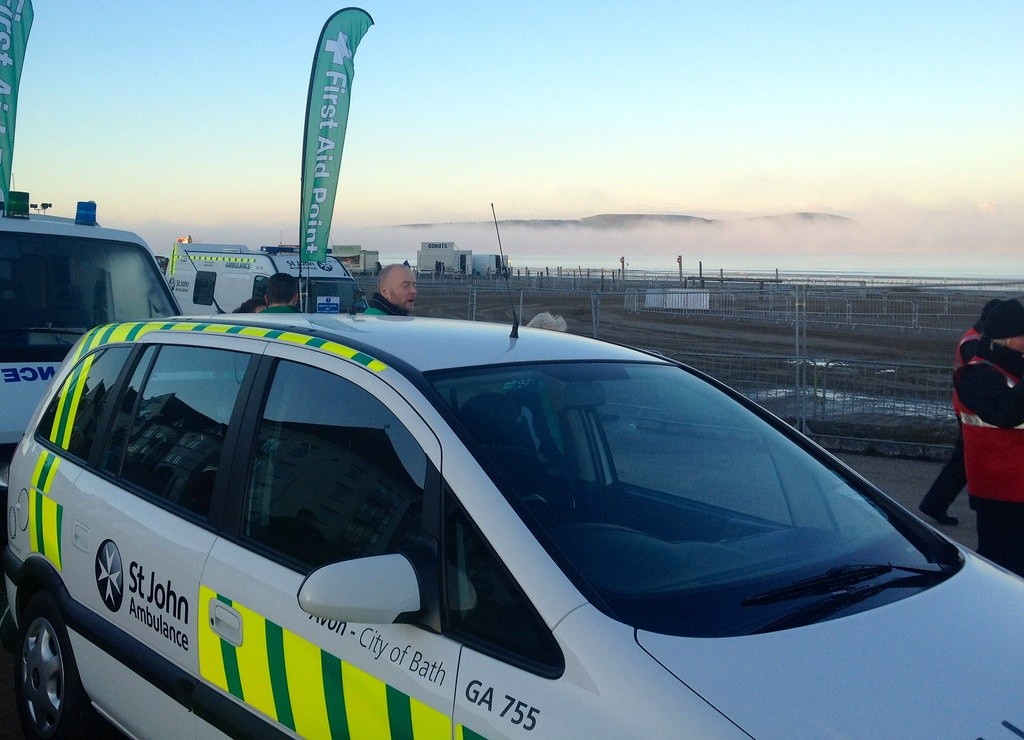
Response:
[982,299,1024,340]
[973,299,1003,333]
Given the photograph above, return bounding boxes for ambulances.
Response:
[158,244,373,314]
[4,312,1024,740]
[0,206,185,444]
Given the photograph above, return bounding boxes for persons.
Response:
[376,261,383,277]
[403,260,410,269]
[435,261,445,279]
[363,263,417,315]
[952,299,1024,580]
[919,299,1003,527]
[232,297,268,313]
[258,273,303,313]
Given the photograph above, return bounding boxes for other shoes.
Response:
[919,499,959,526]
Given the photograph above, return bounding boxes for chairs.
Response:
[460,390,578,527]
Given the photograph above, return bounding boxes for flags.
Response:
[299,7,375,264]
[0,0,34,216]
